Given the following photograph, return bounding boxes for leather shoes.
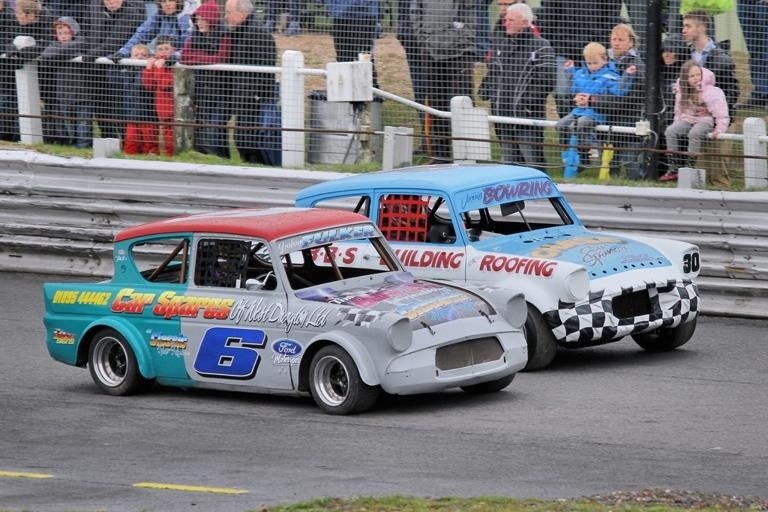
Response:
[658,172,678,182]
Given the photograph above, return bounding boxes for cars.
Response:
[42,203,532,418]
[288,157,706,375]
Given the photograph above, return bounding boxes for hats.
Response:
[57,16,81,37]
